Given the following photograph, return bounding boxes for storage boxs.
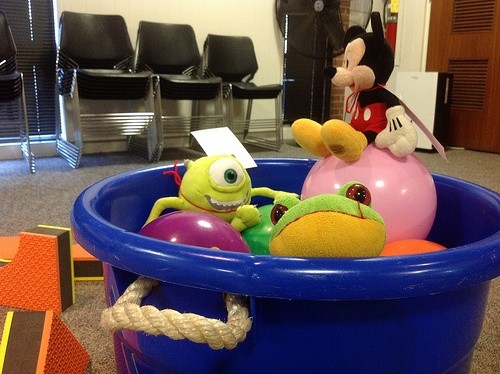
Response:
[70,158,500,374]
[394,70,455,153]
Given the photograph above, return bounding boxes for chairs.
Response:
[0,0,282,174]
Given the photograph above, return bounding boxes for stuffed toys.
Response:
[270,181,387,258]
[142,154,299,232]
[291,12,418,162]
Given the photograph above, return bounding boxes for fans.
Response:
[275,0,373,124]
